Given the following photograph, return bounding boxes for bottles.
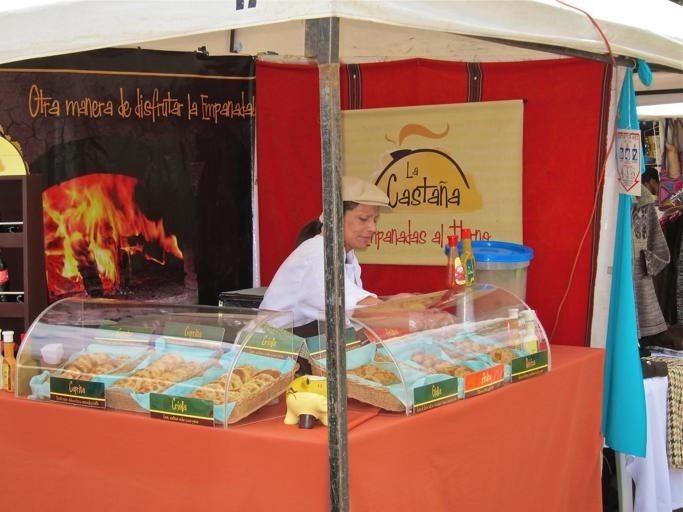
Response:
[507,307,539,354]
[460,229,477,286]
[445,235,466,292]
[17,333,38,396]
[0,331,16,393]
[0,253,10,302]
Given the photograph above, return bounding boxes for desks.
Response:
[613,352,683,511]
[1,341,607,512]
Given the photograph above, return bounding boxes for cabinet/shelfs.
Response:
[1,174,44,345]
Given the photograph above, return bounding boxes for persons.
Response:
[254,175,459,341]
[640,165,662,195]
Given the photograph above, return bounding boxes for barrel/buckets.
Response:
[445,241,534,301]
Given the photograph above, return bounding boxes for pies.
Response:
[346,337,518,386]
[56,351,281,405]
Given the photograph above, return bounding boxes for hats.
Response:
[340,175,393,215]
